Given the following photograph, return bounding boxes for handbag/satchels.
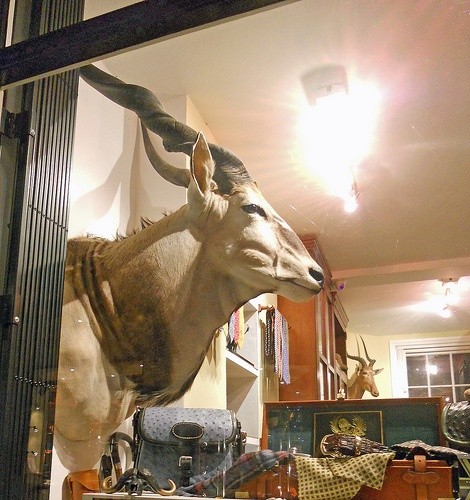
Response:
[132,406,246,491]
[441,400,470,448]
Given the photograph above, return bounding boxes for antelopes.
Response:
[52,64,324,473]
[346,335,385,399]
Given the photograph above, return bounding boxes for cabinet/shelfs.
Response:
[180,300,266,454]
[278,237,349,400]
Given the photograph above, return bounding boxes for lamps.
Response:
[305,66,363,217]
[428,278,462,319]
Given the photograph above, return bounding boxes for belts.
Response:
[315,433,392,459]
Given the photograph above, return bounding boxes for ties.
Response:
[265,308,290,385]
[223,306,244,352]
